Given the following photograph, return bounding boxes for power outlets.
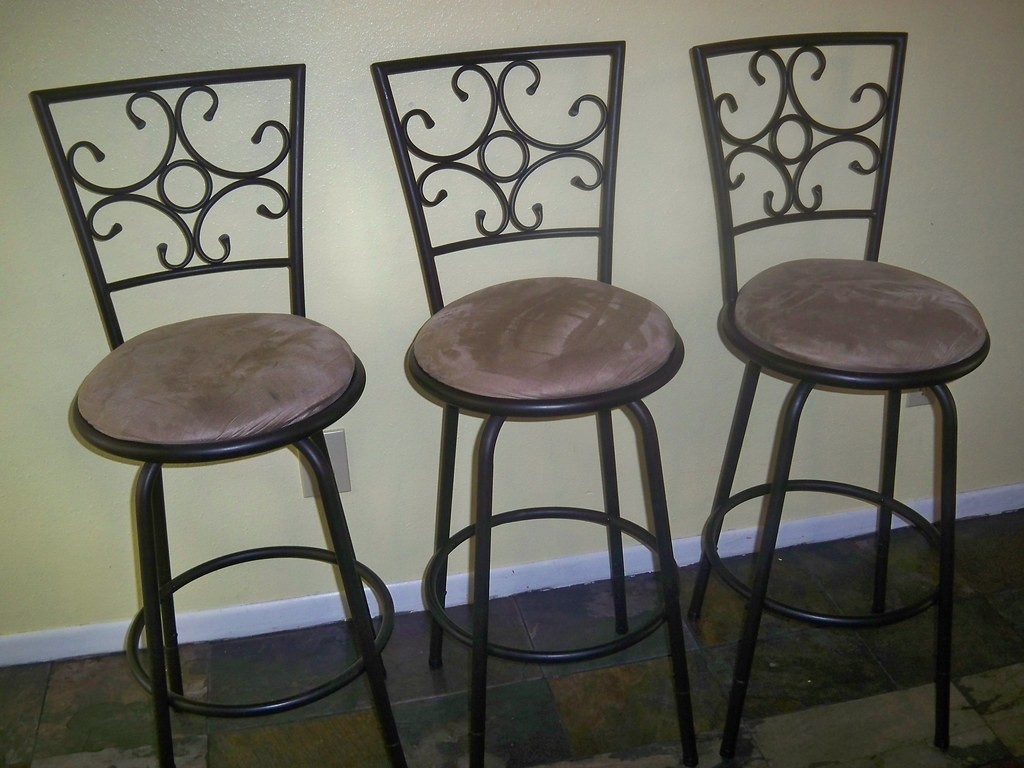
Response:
[298,429,351,498]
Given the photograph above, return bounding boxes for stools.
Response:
[29,63,407,768]
[369,40,699,768]
[688,32,991,762]
